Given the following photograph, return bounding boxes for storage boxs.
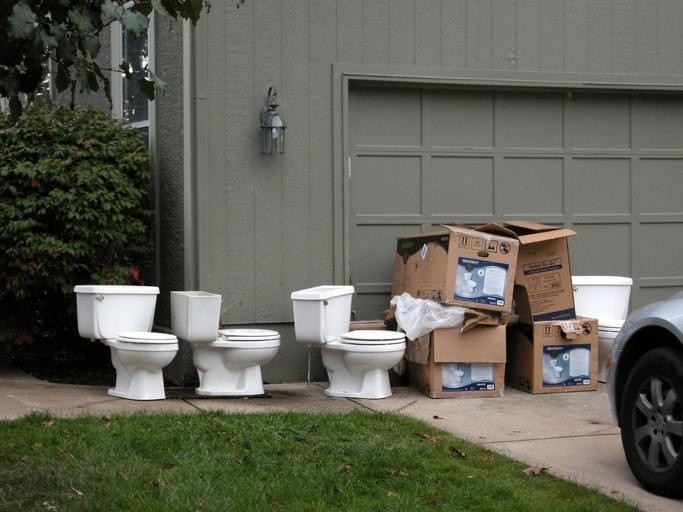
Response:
[505,315,598,395]
[465,220,577,324]
[409,324,507,399]
[391,223,519,313]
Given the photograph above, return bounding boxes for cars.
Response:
[606,286,681,501]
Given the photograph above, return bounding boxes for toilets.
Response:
[73,285,178,400]
[570,275,633,382]
[290,284,407,399]
[171,289,282,396]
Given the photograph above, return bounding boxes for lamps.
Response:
[260,87,287,155]
